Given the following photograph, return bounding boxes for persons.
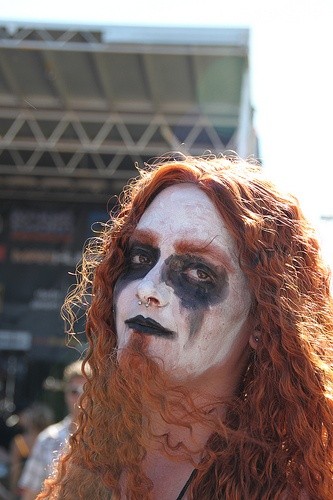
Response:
[19,360,94,500]
[9,402,52,494]
[36,157,333,500]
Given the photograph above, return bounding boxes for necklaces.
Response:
[176,460,202,500]
